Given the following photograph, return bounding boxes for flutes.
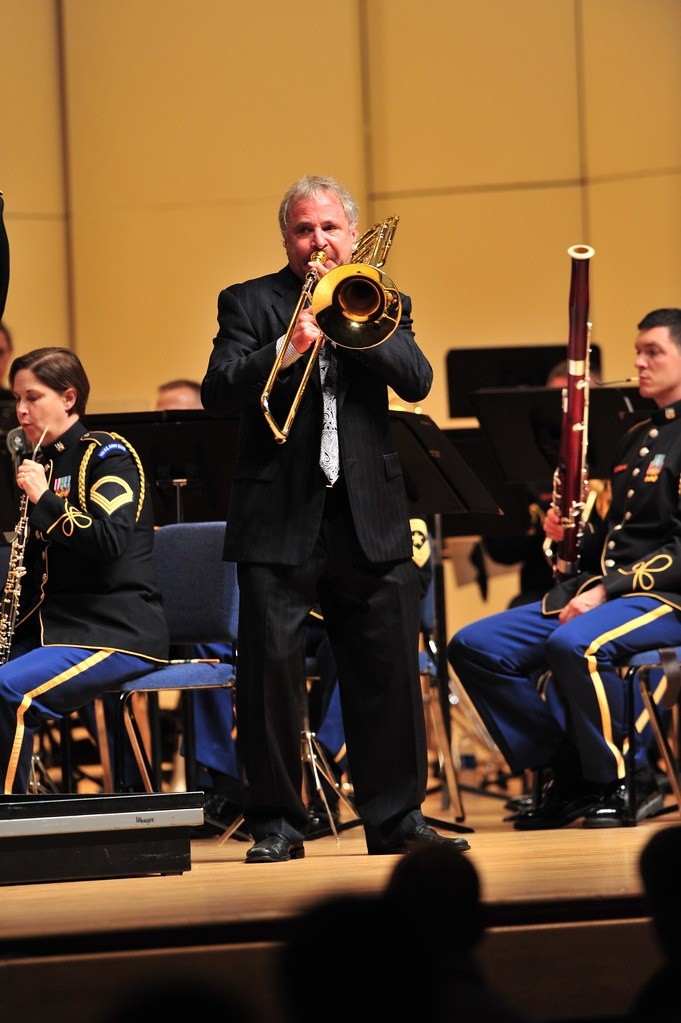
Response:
[544,244,604,587]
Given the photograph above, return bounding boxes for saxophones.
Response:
[0,423,51,669]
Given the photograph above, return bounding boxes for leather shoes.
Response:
[584,765,663,828]
[377,823,470,853]
[514,780,619,829]
[246,833,304,862]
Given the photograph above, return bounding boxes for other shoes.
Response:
[301,809,343,841]
[192,797,243,837]
[504,794,533,811]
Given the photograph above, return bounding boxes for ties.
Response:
[318,339,343,486]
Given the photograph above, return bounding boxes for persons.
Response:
[625,824,681,1023]
[0,321,16,402]
[155,380,343,843]
[0,347,171,795]
[449,307,681,829]
[269,842,487,1023]
[202,176,471,864]
[508,361,672,796]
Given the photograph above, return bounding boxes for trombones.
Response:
[260,211,401,447]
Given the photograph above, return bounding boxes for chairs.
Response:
[536,647,681,808]
[94,521,239,792]
[306,578,465,822]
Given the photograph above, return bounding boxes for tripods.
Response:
[214,409,544,846]
